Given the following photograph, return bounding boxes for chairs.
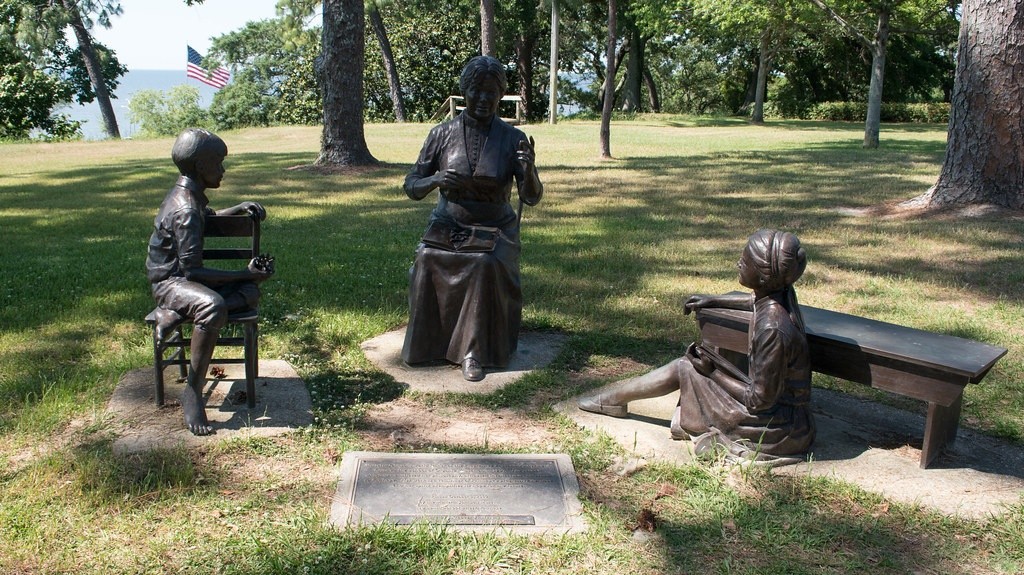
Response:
[144,207,262,409]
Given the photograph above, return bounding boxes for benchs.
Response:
[696,290,1009,469]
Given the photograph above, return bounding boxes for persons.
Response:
[144,127,275,437]
[576,229,819,467]
[402,55,542,381]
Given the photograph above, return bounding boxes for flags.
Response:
[187,45,230,88]
